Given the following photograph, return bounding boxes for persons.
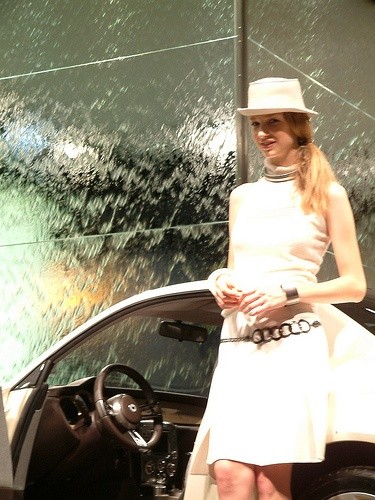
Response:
[205,76,374,500]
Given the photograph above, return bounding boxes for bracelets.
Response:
[279,278,300,307]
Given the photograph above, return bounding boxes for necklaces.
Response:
[262,164,298,182]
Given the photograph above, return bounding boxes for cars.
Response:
[0,279,375,500]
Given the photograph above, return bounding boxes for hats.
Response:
[236,76,318,117]
